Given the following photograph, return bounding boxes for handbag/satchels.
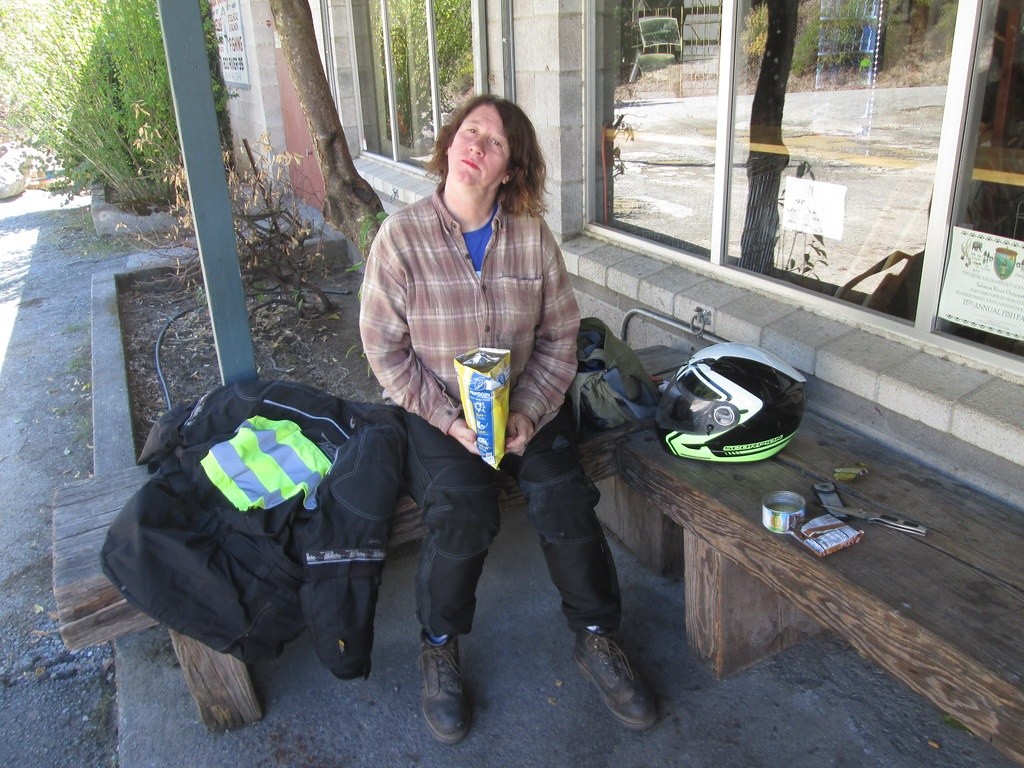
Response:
[565,318,662,435]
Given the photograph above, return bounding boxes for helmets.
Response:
[654,342,807,463]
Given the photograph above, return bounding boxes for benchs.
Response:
[614,397,1024,768]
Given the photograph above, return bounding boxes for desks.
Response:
[52,346,694,733]
[606,128,1024,187]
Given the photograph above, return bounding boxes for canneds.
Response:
[762,490,807,534]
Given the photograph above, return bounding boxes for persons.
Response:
[358,94,658,743]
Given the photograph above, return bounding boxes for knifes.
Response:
[821,503,928,537]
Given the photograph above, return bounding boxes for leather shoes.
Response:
[416,628,472,743]
[569,626,657,730]
